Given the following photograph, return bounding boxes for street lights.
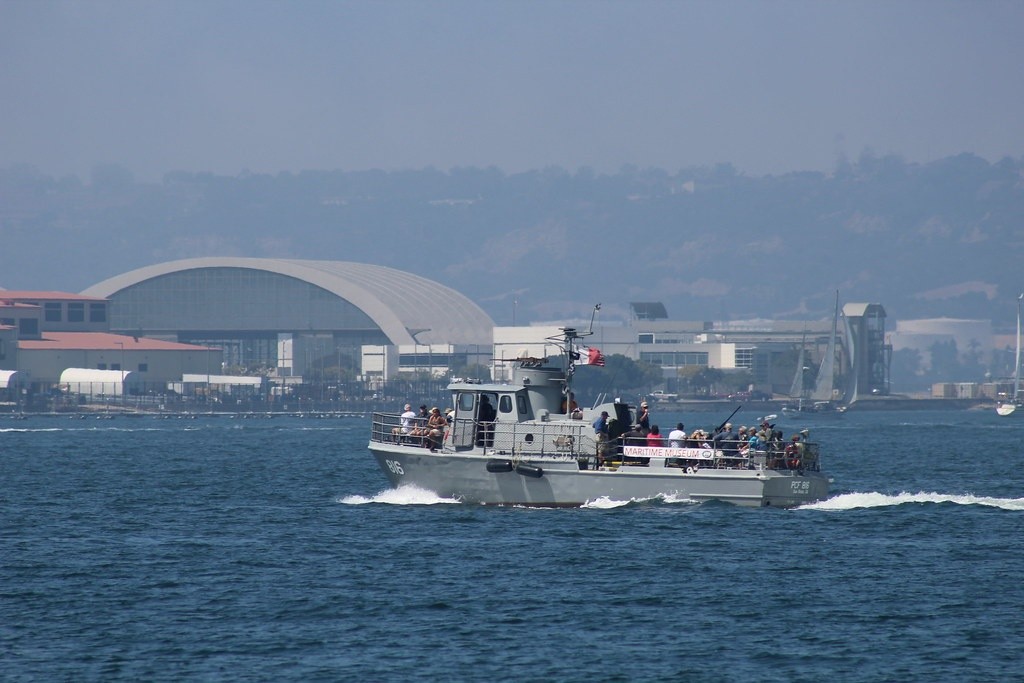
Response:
[803,365,810,414]
[114,340,126,409]
[199,342,210,410]
[10,339,21,413]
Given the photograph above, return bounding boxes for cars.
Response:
[727,389,774,402]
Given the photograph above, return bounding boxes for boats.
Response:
[366,302,828,510]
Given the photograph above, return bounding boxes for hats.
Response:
[760,421,769,427]
[641,402,648,407]
[404,404,411,410]
[443,408,452,413]
[602,411,610,417]
[419,405,426,409]
[429,407,438,414]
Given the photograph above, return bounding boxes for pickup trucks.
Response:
[647,390,679,403]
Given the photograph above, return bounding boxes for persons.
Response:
[592,402,663,467]
[667,422,803,470]
[477,395,494,446]
[392,404,452,448]
[562,393,577,413]
